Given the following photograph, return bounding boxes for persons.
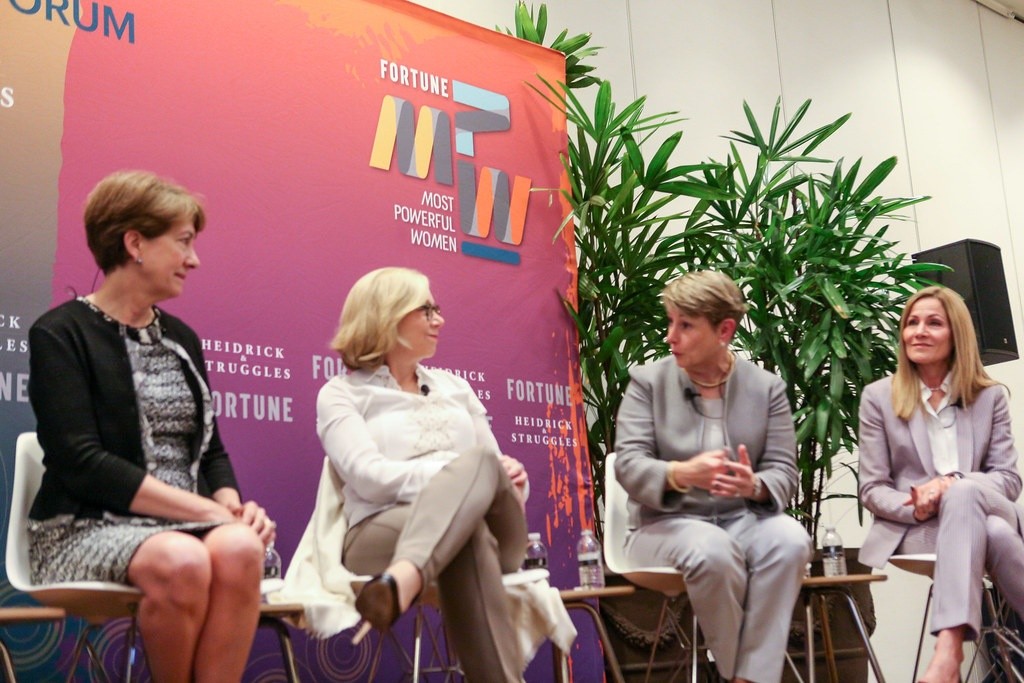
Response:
[614,269,811,683]
[24,169,277,683]
[313,267,531,683]
[859,287,1023,683]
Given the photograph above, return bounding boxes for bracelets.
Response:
[750,476,761,500]
[667,460,692,494]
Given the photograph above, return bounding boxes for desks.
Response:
[799,571,888,682]
[551,585,641,680]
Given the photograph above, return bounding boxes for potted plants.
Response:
[499,0,955,682]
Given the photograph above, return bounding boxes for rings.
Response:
[929,489,935,494]
[733,487,742,497]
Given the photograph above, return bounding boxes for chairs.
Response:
[0,429,1024,682]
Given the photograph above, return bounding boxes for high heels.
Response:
[351,576,400,646]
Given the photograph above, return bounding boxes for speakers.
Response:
[912,239,1019,367]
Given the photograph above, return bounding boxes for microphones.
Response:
[126,327,140,342]
[684,387,696,406]
[956,397,962,409]
[421,385,430,396]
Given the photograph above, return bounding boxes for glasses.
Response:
[415,305,440,321]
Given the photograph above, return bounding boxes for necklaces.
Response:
[929,387,942,391]
[689,376,728,388]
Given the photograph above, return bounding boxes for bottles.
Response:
[263,541,282,579]
[821,528,847,577]
[523,532,549,570]
[576,529,605,590]
